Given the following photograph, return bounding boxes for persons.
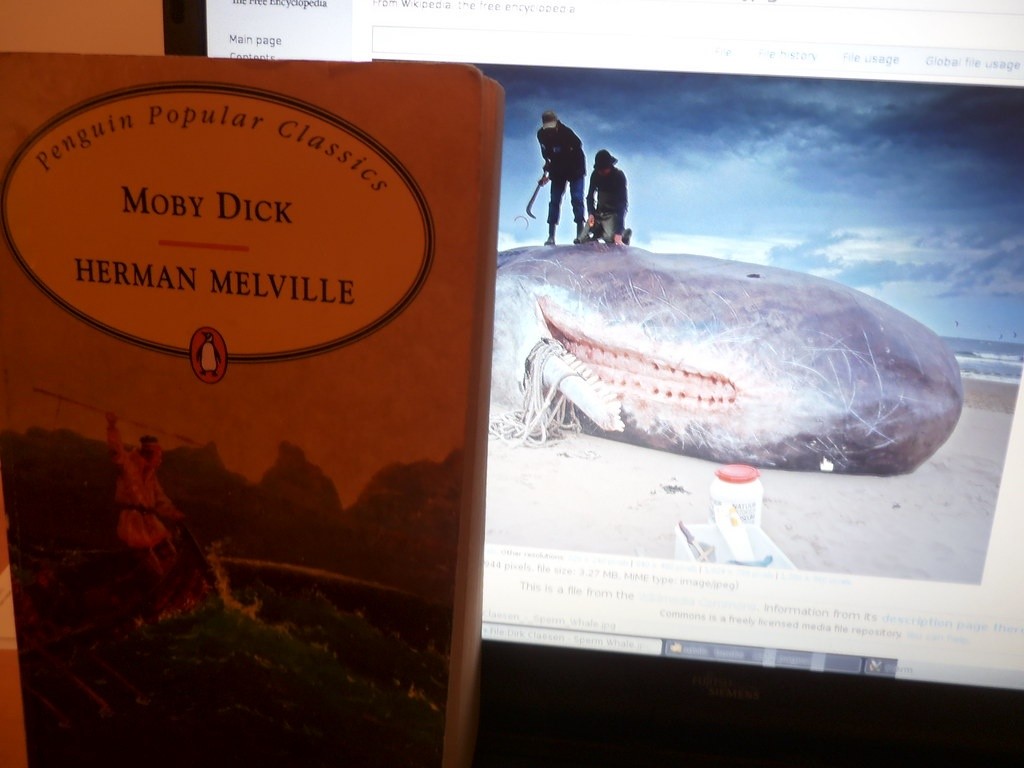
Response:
[537,111,633,248]
[107,411,185,614]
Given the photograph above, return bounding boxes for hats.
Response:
[541,111,558,130]
[593,149,619,169]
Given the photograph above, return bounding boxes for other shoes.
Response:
[623,228,632,245]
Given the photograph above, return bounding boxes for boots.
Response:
[544,224,557,245]
[573,223,590,244]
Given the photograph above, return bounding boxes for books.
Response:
[1,52,504,767]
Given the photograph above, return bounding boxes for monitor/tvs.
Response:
[160,0,1024,768]
